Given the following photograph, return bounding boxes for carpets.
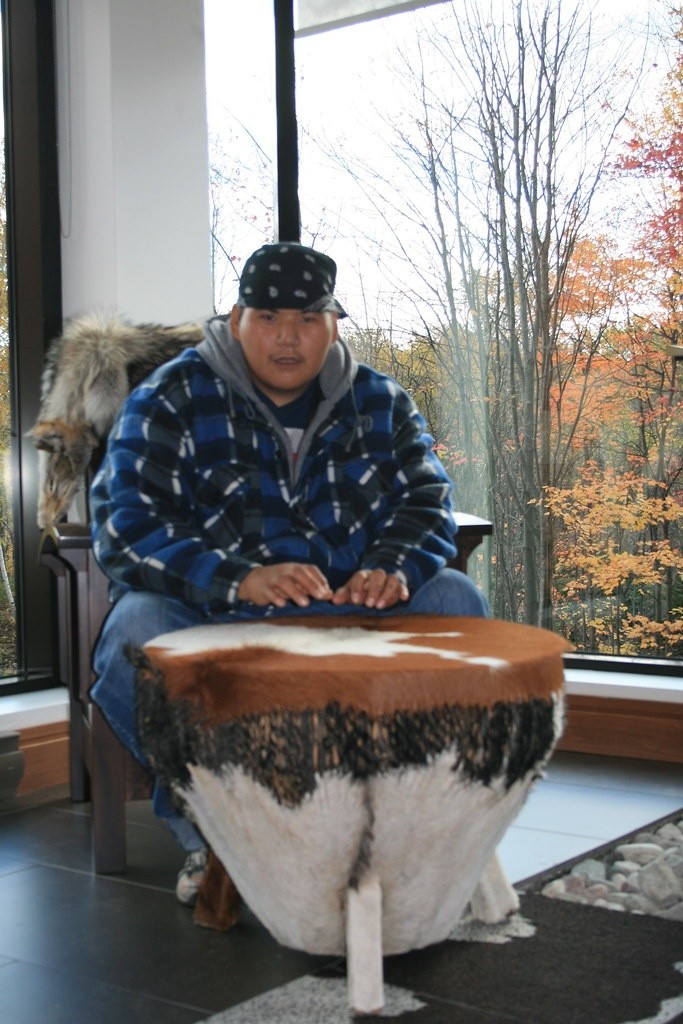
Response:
[193,891,683,1024]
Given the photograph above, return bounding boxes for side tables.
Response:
[141,613,574,1024]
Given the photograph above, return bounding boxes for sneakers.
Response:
[176,848,209,906]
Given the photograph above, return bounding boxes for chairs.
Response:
[39,319,493,878]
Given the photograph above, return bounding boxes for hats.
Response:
[237,244,347,317]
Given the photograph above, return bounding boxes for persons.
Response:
[85,243,493,903]
